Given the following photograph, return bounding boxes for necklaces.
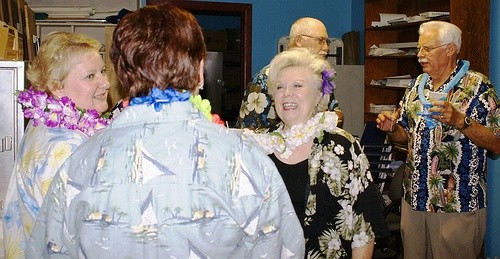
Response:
[123,87,225,127]
[416,59,470,129]
[13,85,110,137]
[272,111,338,159]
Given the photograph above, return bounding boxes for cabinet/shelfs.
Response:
[363,0,490,125]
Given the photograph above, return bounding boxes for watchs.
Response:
[460,116,471,130]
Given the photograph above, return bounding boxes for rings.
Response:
[441,116,443,119]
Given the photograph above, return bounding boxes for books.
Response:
[369,103,397,114]
[383,75,413,87]
[371,11,450,27]
[379,42,418,48]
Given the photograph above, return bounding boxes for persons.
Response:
[235,17,345,133]
[264,47,376,259]
[24,2,306,259]
[376,20,500,259]
[2,30,110,259]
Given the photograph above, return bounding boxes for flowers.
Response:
[322,69,336,96]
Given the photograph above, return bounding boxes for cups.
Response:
[429,92,449,114]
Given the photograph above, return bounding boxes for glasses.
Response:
[414,44,447,54]
[302,35,330,47]
